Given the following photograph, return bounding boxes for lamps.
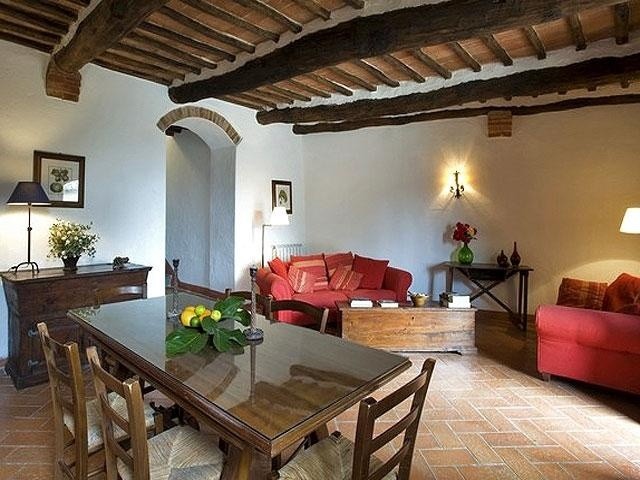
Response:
[616,208,640,235]
[256,206,289,268]
[7,181,53,274]
[447,168,466,198]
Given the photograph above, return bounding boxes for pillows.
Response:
[323,251,354,280]
[267,257,287,279]
[560,277,607,311]
[291,252,330,291]
[352,253,389,290]
[602,272,640,316]
[327,263,364,292]
[287,265,315,294]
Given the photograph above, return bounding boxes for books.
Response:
[443,291,470,303]
[438,294,472,309]
[350,297,374,309]
[376,300,399,309]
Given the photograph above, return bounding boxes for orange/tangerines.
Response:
[190,317,200,328]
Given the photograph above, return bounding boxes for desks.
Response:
[444,259,533,333]
[66,290,413,479]
[334,297,480,355]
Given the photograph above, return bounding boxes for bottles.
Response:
[496,250,508,266]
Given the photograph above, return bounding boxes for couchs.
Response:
[258,251,412,328]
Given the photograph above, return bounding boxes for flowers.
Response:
[47,215,96,257]
[448,218,479,243]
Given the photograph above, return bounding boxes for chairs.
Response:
[223,286,273,316]
[81,341,225,480]
[535,271,640,396]
[276,356,439,478]
[30,317,166,480]
[256,292,331,470]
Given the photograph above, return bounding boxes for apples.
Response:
[211,310,221,322]
[194,304,205,315]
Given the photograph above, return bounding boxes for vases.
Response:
[458,242,472,264]
[497,249,507,266]
[62,255,82,271]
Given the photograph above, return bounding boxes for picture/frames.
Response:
[33,149,86,209]
[272,180,293,214]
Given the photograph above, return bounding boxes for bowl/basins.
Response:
[409,295,429,306]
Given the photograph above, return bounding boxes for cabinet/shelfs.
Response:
[2,261,155,391]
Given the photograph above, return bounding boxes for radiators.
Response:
[272,242,304,264]
